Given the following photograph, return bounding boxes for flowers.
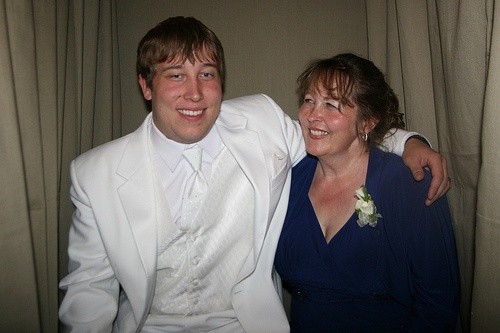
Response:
[352,184,383,228]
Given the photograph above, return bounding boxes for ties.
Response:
[179,146,208,231]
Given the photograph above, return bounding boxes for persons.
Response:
[58,16,452,333]
[274,53,463,333]
